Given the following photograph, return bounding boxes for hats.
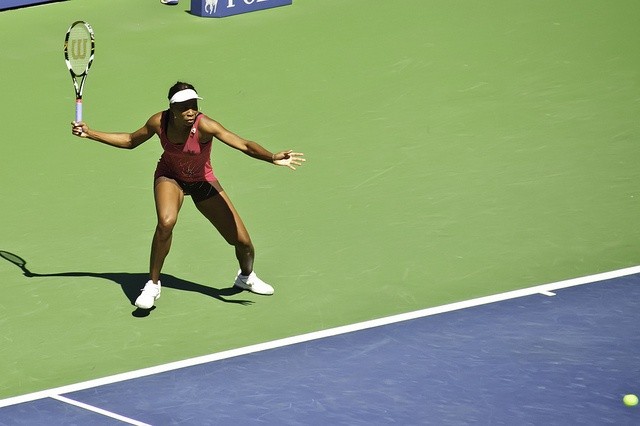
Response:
[169,88,204,103]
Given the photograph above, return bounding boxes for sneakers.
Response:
[135,278,161,309]
[234,268,274,295]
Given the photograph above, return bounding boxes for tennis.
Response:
[623,393,639,407]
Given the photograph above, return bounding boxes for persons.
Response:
[70,80,306,309]
